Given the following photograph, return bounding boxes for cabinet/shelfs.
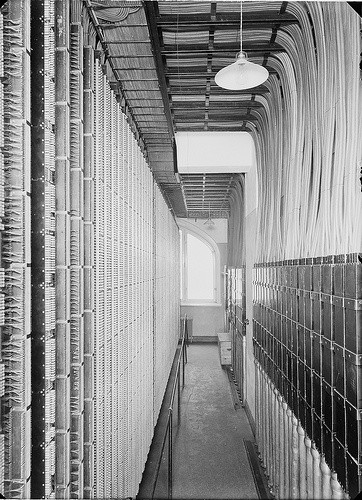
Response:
[218,332,231,366]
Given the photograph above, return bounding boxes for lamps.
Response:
[214,1,269,91]
